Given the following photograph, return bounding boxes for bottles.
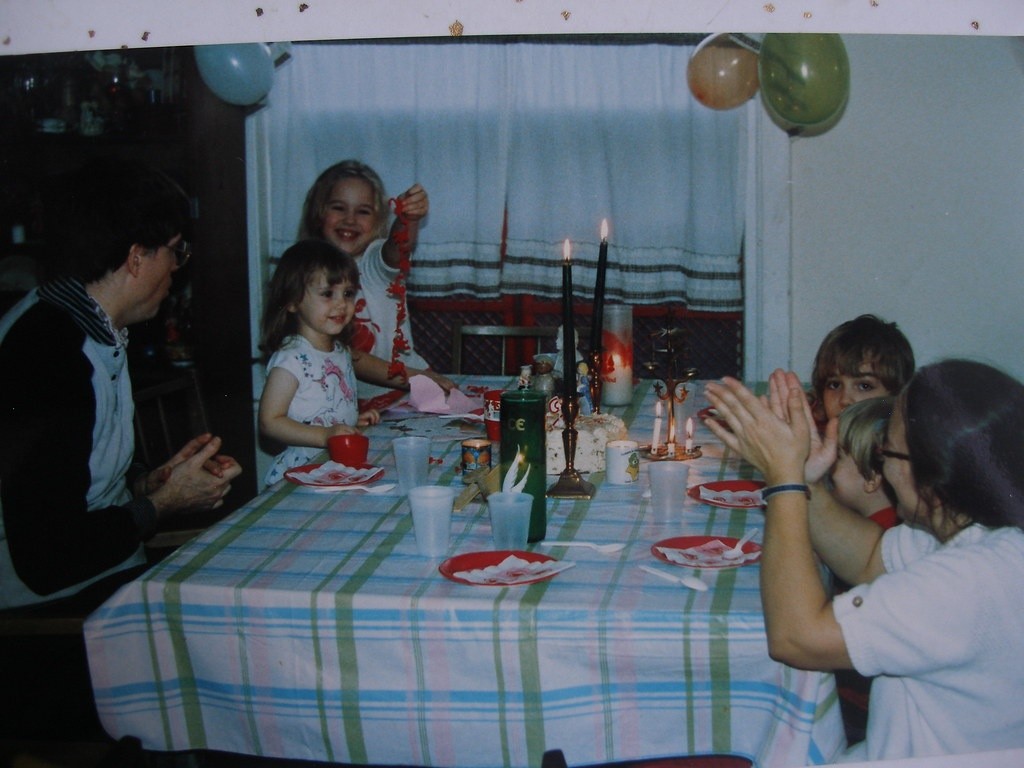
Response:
[517,366,532,391]
[462,440,492,486]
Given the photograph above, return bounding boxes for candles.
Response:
[668,425,675,457]
[686,417,693,455]
[591,218,608,348]
[602,354,632,405]
[562,238,577,395]
[651,402,662,455]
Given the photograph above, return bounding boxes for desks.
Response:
[78,371,848,768]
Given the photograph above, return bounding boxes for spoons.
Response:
[314,484,396,494]
[639,565,708,590]
[439,413,483,422]
[722,528,759,559]
[542,542,628,554]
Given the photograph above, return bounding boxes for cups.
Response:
[486,492,534,551]
[606,440,639,485]
[484,390,512,444]
[392,436,431,494]
[647,462,690,520]
[328,435,369,466]
[409,486,454,556]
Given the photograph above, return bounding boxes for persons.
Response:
[298,160,431,408]
[0,165,242,620]
[812,315,915,419]
[260,240,381,484]
[705,360,1024,762]
[826,398,901,528]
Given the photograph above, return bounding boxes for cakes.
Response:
[542,410,630,474]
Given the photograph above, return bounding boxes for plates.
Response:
[688,480,768,508]
[698,406,731,428]
[651,535,764,569]
[439,550,558,586]
[464,408,484,423]
[284,463,384,488]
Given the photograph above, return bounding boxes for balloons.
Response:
[759,34,849,123]
[691,33,759,110]
[193,43,275,108]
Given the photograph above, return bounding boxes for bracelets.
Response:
[762,484,810,499]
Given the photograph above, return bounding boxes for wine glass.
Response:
[102,65,126,119]
[16,71,46,138]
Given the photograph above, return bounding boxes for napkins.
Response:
[288,459,384,485]
[698,483,768,506]
[656,538,762,567]
[453,553,576,585]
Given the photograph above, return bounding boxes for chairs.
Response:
[448,317,591,376]
[1,527,201,768]
[129,370,212,468]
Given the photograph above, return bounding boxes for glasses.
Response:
[876,429,914,462]
[151,237,194,267]
